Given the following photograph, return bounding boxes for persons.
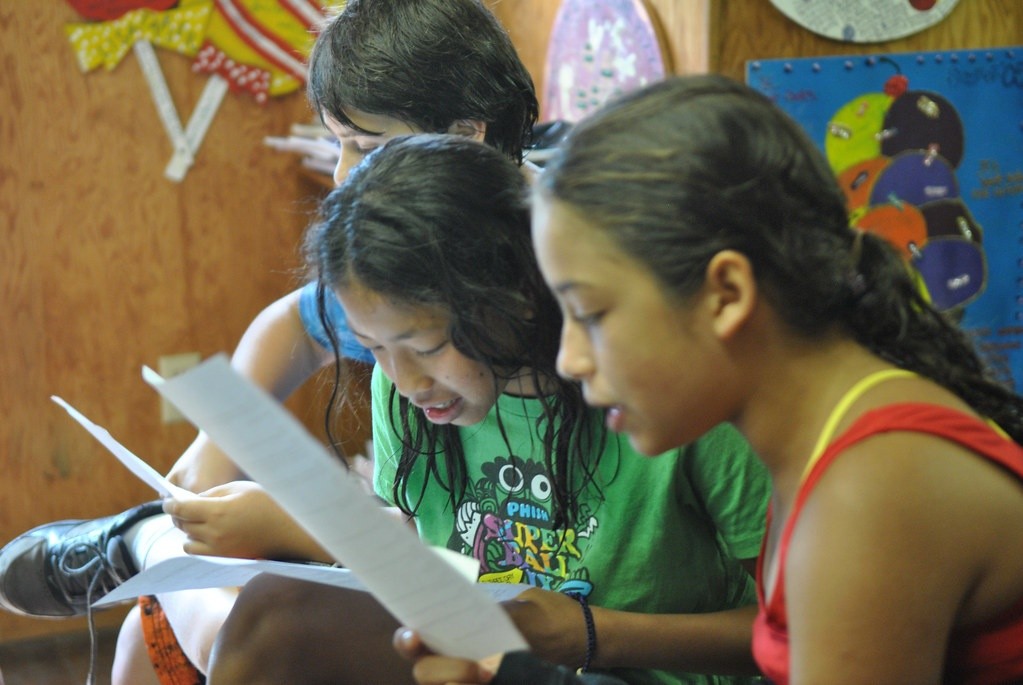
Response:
[392,74,1022,685]
[0,0,778,685]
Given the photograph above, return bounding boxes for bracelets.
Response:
[566,592,597,676]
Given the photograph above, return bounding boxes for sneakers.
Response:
[0,500,163,685]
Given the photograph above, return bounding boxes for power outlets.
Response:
[158,351,202,427]
